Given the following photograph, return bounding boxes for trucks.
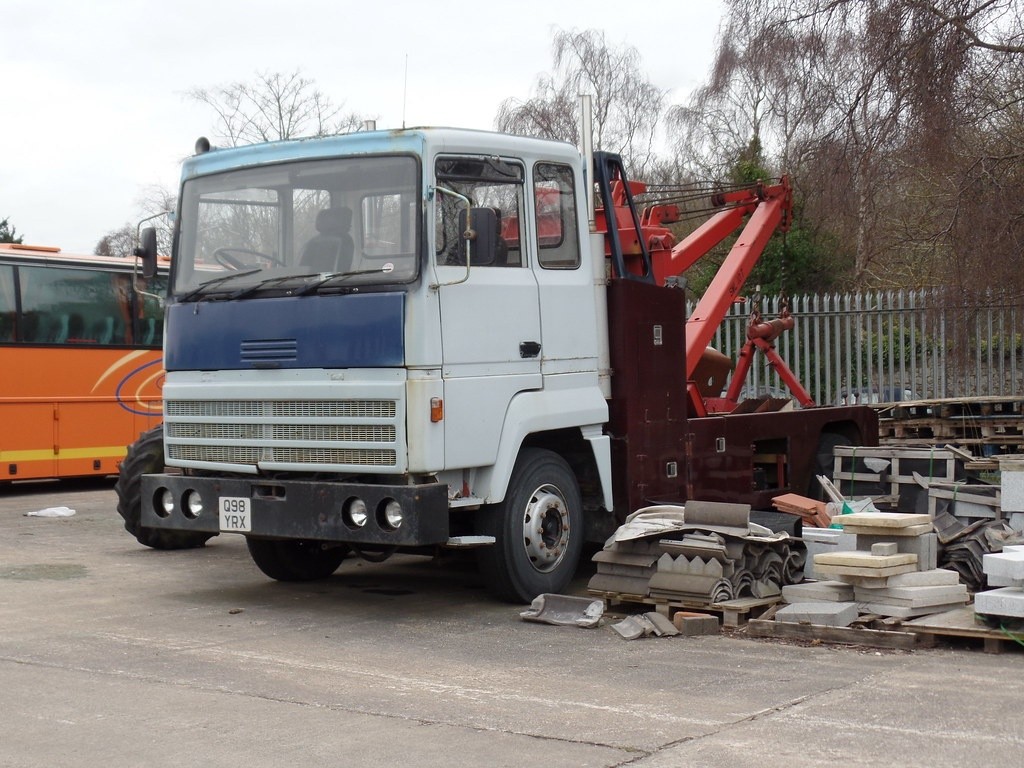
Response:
[132,89,882,607]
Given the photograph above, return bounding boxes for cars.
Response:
[735,385,934,420]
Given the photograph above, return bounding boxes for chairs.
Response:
[139,316,157,346]
[91,317,118,345]
[301,206,356,273]
[446,206,510,268]
[43,314,69,343]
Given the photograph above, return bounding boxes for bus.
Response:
[0,241,276,494]
